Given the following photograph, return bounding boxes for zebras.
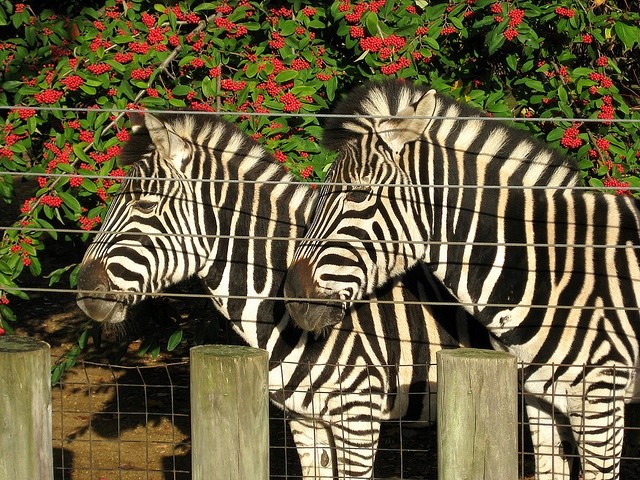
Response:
[77,107,494,480]
[283,77,639,480]
